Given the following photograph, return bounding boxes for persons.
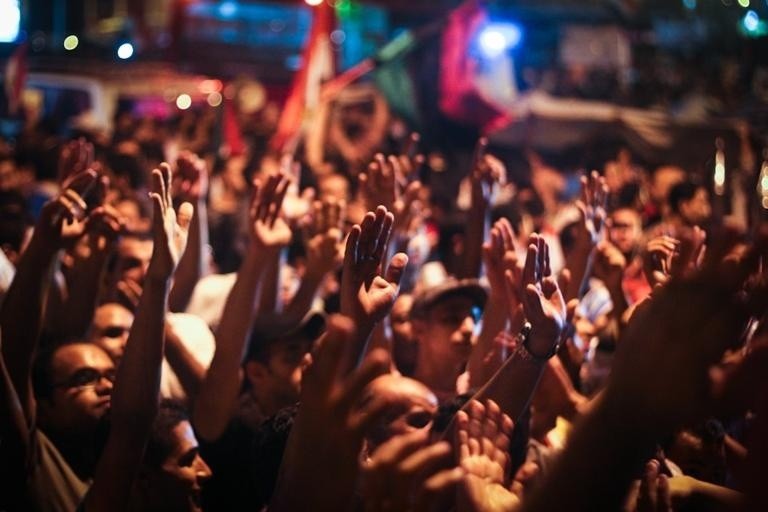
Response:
[0,0,768,511]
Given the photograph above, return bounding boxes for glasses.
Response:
[51,366,117,391]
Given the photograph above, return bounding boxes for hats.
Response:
[242,313,327,363]
[408,279,487,324]
